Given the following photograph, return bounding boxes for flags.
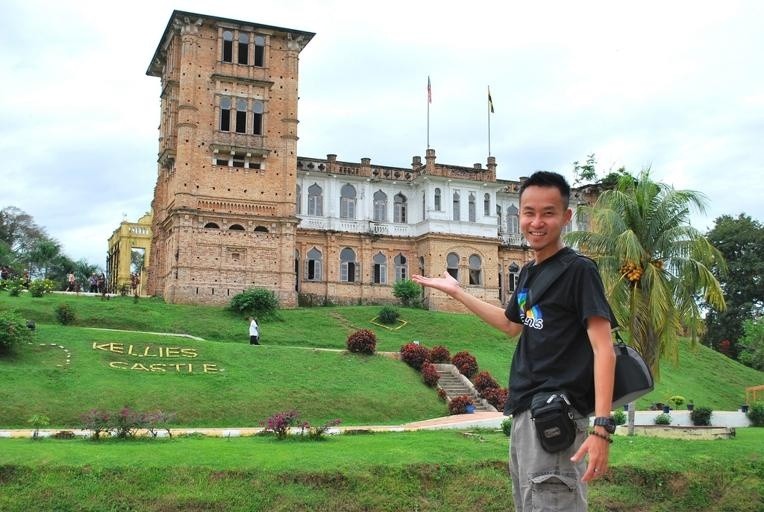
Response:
[488,87,494,113]
[427,76,432,101]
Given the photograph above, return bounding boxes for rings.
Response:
[594,467,599,473]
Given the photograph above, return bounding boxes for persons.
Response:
[247,315,261,345]
[409,170,620,512]
[0,263,140,295]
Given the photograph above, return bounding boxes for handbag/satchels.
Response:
[530,390,578,453]
[583,342,655,417]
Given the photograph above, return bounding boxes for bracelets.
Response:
[589,431,614,445]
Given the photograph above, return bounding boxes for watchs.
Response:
[594,416,616,434]
